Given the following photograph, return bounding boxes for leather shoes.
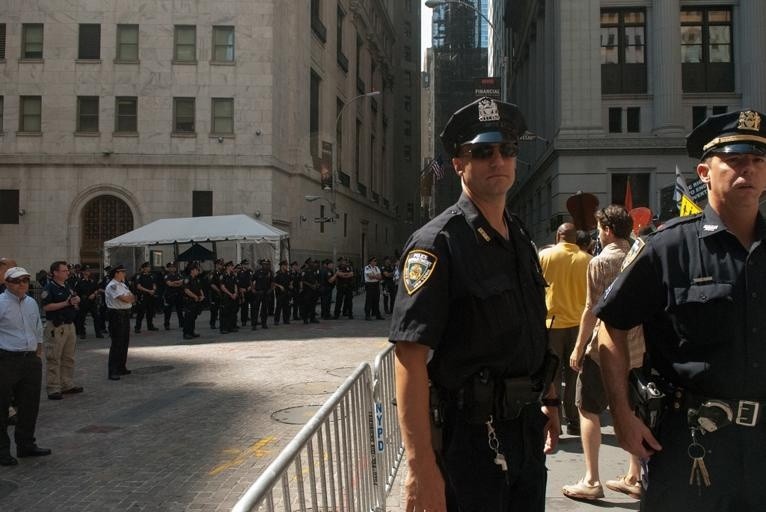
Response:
[108,370,130,381]
[565,418,581,434]
[209,313,292,334]
[18,446,51,457]
[295,309,392,324]
[135,323,168,334]
[607,476,643,499]
[565,480,604,501]
[183,333,199,338]
[1,456,17,466]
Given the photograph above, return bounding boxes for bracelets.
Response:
[542,395,562,409]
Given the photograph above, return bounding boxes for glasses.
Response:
[459,143,518,160]
[8,277,29,284]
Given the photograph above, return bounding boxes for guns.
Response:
[427,378,443,451]
[628,368,666,450]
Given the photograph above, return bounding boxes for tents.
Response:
[103,214,289,275]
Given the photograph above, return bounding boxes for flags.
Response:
[672,163,694,212]
[624,179,633,212]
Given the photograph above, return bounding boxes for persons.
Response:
[0,265,50,465]
[590,108,764,512]
[539,203,646,503]
[66,256,356,339]
[364,256,401,321]
[387,93,564,512]
[41,261,83,399]
[0,257,17,293]
[104,264,135,380]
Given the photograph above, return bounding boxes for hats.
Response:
[111,265,127,273]
[166,262,175,267]
[441,97,528,152]
[4,267,30,281]
[278,260,289,266]
[216,258,249,265]
[684,109,765,156]
[260,259,270,265]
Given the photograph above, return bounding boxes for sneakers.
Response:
[64,387,82,394]
[49,393,63,400]
[9,414,18,423]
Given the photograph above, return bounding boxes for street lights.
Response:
[425,0,506,105]
[302,91,383,280]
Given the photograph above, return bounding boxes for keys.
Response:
[688,454,713,487]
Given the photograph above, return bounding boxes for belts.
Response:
[658,378,764,429]
